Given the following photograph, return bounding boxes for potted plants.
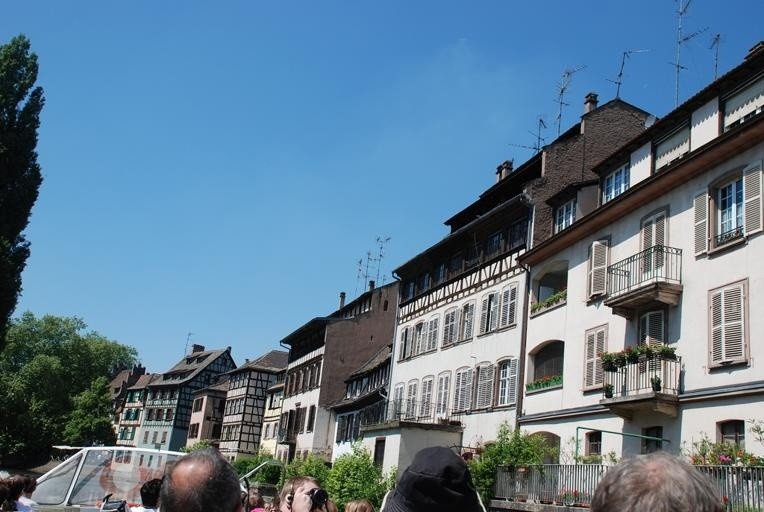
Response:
[526,376,563,391]
[600,344,676,372]
[514,488,593,507]
[600,383,614,398]
[651,376,661,392]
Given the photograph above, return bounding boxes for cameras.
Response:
[305,487,328,509]
[100,494,126,512]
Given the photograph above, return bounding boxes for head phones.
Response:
[288,476,300,500]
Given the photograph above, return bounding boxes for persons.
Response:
[249,475,376,512]
[589,450,726,512]
[129,479,162,512]
[0,471,41,512]
[161,447,243,512]
